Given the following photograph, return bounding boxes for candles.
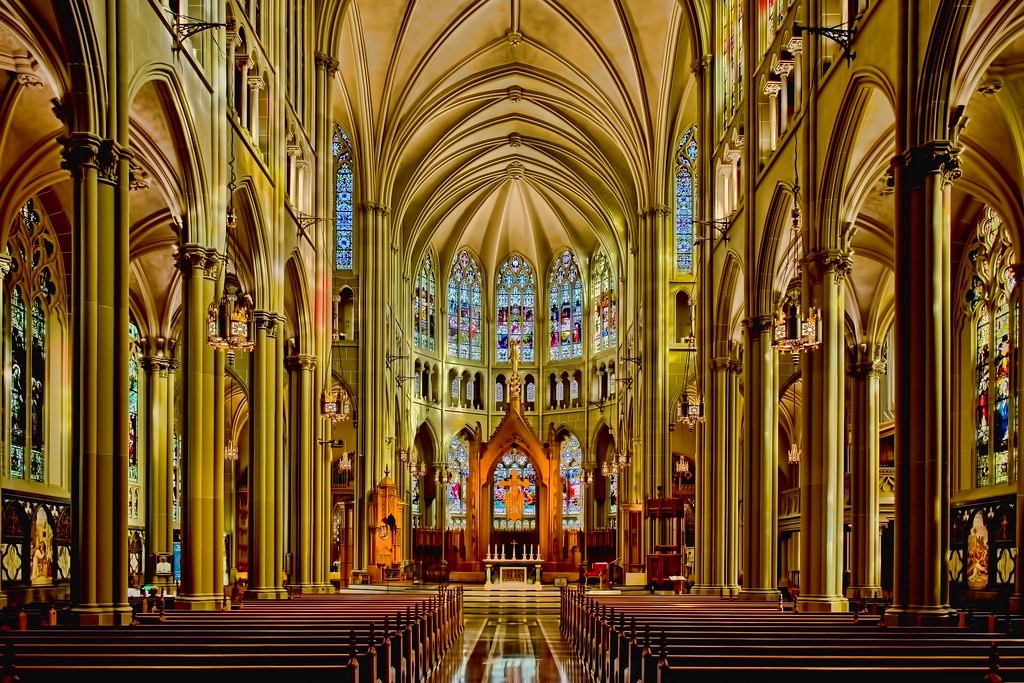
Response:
[502,544,504,554]
[538,546,540,554]
[488,544,490,554]
[495,544,497,554]
[531,544,533,554]
[524,544,526,554]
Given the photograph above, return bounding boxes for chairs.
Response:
[584,562,609,589]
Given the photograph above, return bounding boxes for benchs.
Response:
[0,583,464,683]
[558,585,1024,683]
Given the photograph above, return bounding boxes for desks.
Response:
[482,559,545,592]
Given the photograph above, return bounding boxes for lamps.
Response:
[165,8,256,366]
[676,421,688,473]
[297,211,351,435]
[771,13,861,371]
[788,384,802,464]
[676,209,735,430]
[224,377,238,461]
[601,356,642,477]
[338,422,353,474]
[400,448,452,485]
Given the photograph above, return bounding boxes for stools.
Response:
[351,572,370,585]
